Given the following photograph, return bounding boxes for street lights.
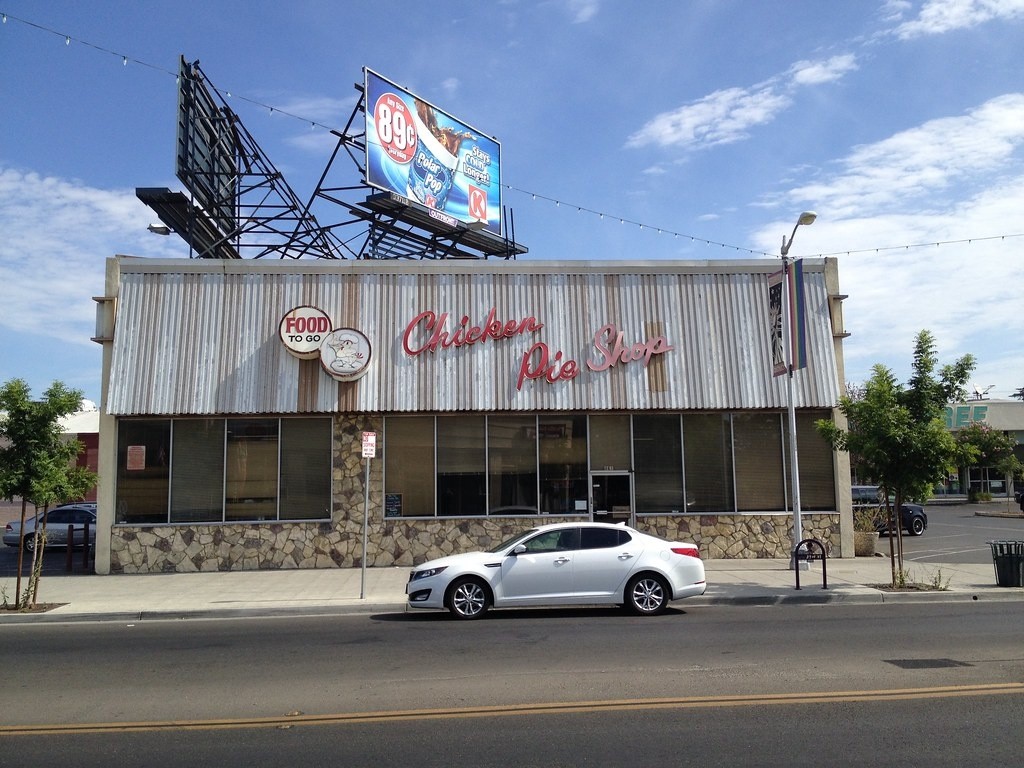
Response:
[781,210,818,571]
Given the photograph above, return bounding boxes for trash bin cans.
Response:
[985,539,1024,588]
[1015,493,1021,503]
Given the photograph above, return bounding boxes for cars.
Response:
[2,503,97,554]
[403,521,707,620]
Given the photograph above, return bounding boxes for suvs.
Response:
[851,485,928,537]
